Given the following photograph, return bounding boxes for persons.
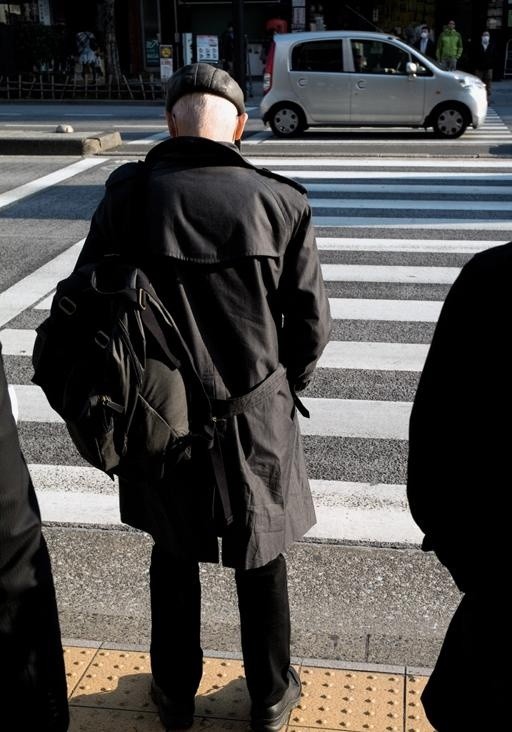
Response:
[412,25,439,66]
[70,62,334,729]
[0,345,70,732]
[470,30,494,107]
[408,240,512,732]
[437,19,464,71]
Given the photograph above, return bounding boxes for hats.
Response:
[165,63,244,150]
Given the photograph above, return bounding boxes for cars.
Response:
[260,30,489,140]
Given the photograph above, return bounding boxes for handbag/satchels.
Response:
[32,177,229,485]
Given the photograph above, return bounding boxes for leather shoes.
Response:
[250,664,301,732]
[150,676,195,732]
